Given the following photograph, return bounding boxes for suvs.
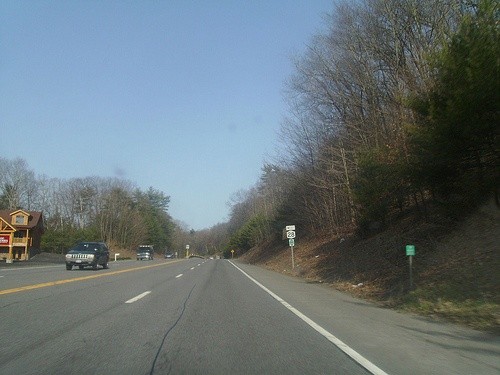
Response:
[66,242,109,270]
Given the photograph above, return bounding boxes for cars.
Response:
[164,253,173,259]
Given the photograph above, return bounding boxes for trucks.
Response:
[137,245,153,260]
